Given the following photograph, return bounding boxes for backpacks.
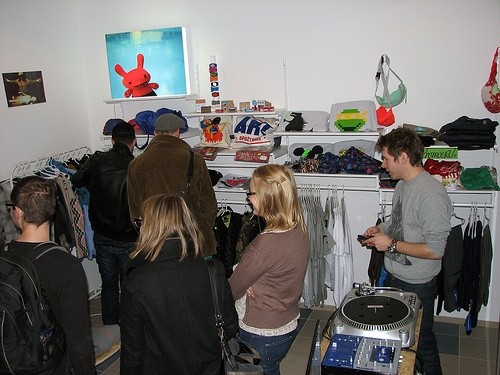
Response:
[0,239,67,375]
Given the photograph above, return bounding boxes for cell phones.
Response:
[358,234,370,240]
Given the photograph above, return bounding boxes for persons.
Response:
[120,194,238,375]
[227,162,311,375]
[71,122,135,326]
[0,176,96,375]
[126,113,240,341]
[6,73,42,93]
[361,128,453,375]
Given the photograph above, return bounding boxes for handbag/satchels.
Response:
[135,111,156,135]
[200,116,230,148]
[129,119,143,135]
[460,165,500,190]
[230,115,279,152]
[339,147,385,175]
[481,47,500,113]
[375,83,407,108]
[376,106,395,126]
[219,340,263,375]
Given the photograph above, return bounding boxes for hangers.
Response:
[9,148,94,188]
[375,196,492,239]
[243,201,254,221]
[293,184,347,214]
[217,198,232,218]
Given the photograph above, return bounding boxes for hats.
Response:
[112,121,137,146]
[103,119,125,135]
[156,114,185,131]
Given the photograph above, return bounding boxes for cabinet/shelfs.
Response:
[102,56,498,209]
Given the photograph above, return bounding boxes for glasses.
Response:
[5,202,15,210]
[134,217,142,229]
[246,189,255,196]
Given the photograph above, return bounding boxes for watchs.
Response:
[388,239,398,252]
